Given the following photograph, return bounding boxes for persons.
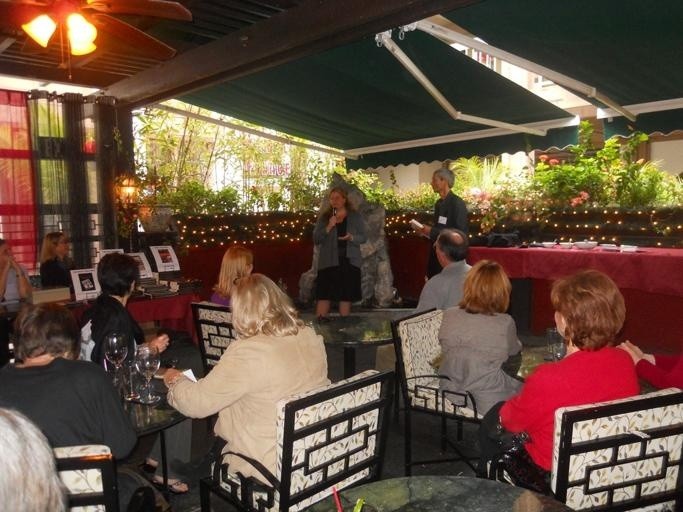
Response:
[1,407,70,511]
[76,253,195,493]
[346,184,398,310]
[312,187,369,316]
[437,258,524,474]
[614,339,683,391]
[210,247,254,304]
[38,231,77,293]
[475,269,641,495]
[413,168,470,282]
[1,302,170,511]
[0,239,33,300]
[162,273,333,490]
[414,228,475,314]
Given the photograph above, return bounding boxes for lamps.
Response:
[19,1,98,58]
[113,173,143,253]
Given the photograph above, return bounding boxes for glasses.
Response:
[433,242,439,250]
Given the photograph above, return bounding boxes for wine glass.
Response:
[134,344,161,404]
[122,336,140,401]
[102,332,128,389]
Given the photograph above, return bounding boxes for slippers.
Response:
[142,457,158,474]
[150,475,191,495]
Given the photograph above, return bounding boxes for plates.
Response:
[574,241,597,249]
[619,245,636,253]
[599,244,616,252]
[558,242,574,249]
[541,242,556,247]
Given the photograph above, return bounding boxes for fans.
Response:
[0,0,193,63]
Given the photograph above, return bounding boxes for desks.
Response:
[470,243,682,353]
[19,279,206,346]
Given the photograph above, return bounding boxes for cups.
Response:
[546,326,569,361]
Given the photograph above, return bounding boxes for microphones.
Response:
[333,207,337,218]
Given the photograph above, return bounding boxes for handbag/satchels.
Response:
[476,400,529,462]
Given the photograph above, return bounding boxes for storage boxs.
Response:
[24,285,71,305]
